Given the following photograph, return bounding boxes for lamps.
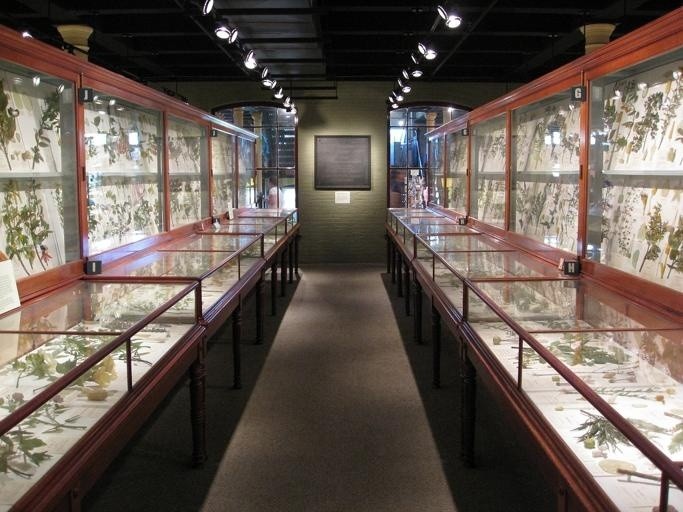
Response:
[188,1,298,115]
[384,1,463,110]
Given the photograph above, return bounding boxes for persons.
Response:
[267,176,281,208]
[391,168,405,207]
